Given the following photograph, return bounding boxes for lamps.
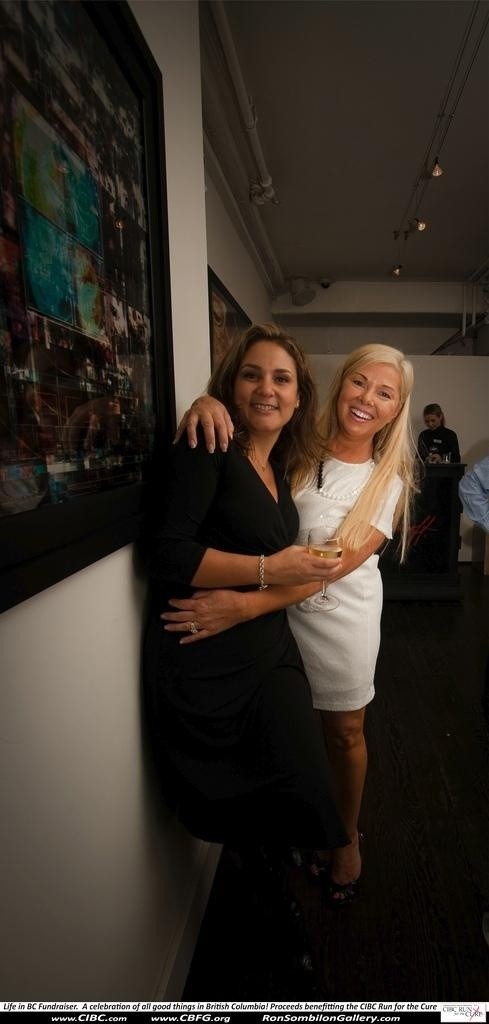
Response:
[392,264,402,276]
[413,216,426,231]
[432,156,443,177]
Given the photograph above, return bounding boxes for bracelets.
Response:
[258,554,268,592]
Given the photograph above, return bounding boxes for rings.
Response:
[189,621,197,633]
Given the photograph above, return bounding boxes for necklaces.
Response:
[249,448,273,474]
[314,457,376,502]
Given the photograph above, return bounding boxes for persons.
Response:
[162,342,426,908]
[136,323,349,1004]
[415,402,460,467]
[456,456,488,537]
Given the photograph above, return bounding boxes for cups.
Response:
[443,453,450,463]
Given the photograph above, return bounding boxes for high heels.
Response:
[310,833,363,911]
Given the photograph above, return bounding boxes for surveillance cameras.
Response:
[320,278,334,289]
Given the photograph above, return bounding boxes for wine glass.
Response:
[308,526,343,612]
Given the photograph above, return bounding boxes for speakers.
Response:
[292,274,315,305]
